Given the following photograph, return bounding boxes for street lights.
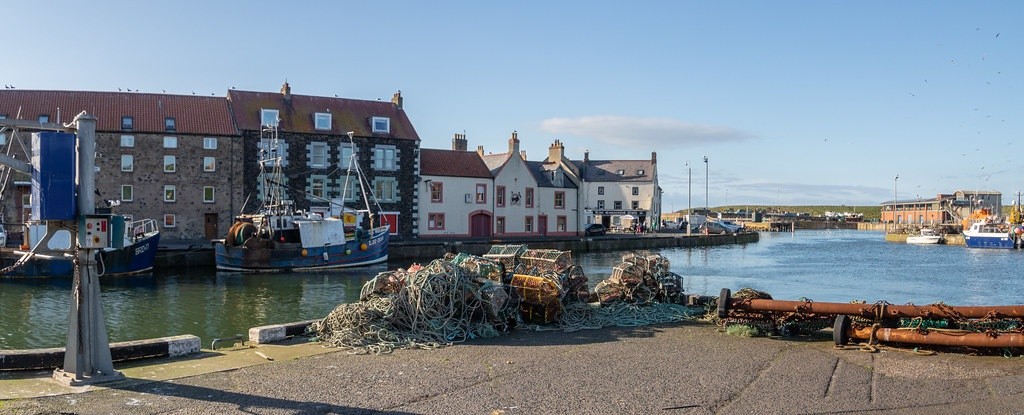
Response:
[703,155,708,220]
[685,162,692,215]
[894,175,899,224]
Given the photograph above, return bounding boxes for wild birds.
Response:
[334,93,381,101]
[232,86,235,90]
[118,86,215,95]
[6,84,16,90]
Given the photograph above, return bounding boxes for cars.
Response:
[698,221,745,234]
[579,223,606,236]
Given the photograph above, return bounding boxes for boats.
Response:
[962,224,1015,249]
[215,130,393,273]
[1,219,162,279]
[907,235,943,244]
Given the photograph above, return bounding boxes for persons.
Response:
[633,219,648,233]
